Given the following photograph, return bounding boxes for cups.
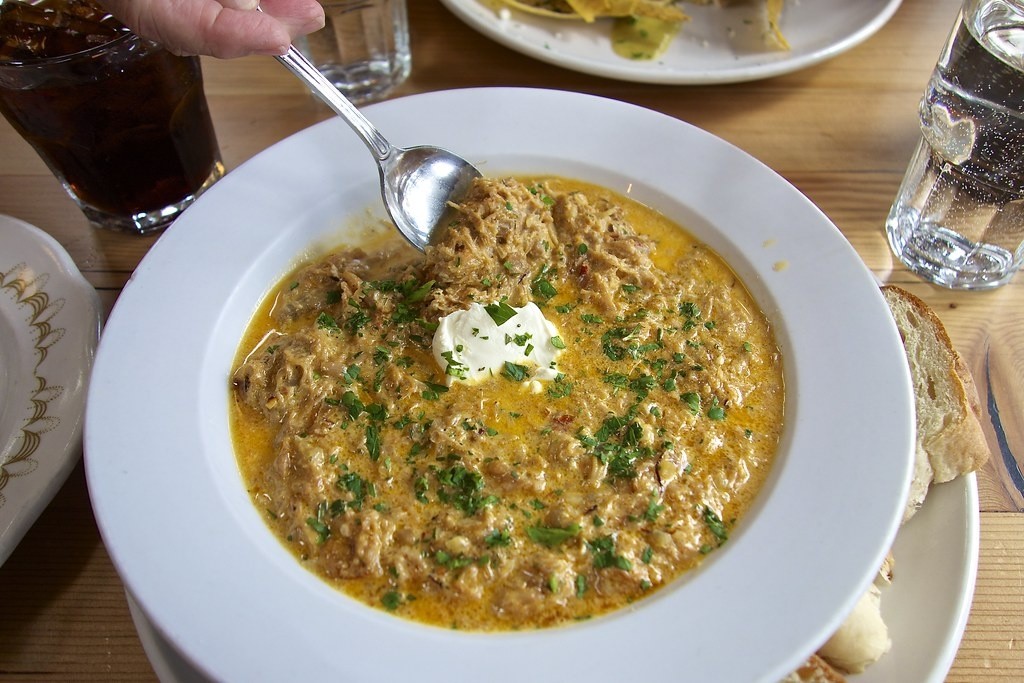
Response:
[290,1,411,104]
[874,3,1024,295]
[2,2,229,232]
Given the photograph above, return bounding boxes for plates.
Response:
[0,210,102,562]
[440,2,899,89]
[121,471,980,683]
[79,92,915,683]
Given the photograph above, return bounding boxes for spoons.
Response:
[254,2,487,251]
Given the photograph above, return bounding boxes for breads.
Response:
[782,549,894,683]
[879,284,989,526]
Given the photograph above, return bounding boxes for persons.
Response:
[92,0,329,62]
